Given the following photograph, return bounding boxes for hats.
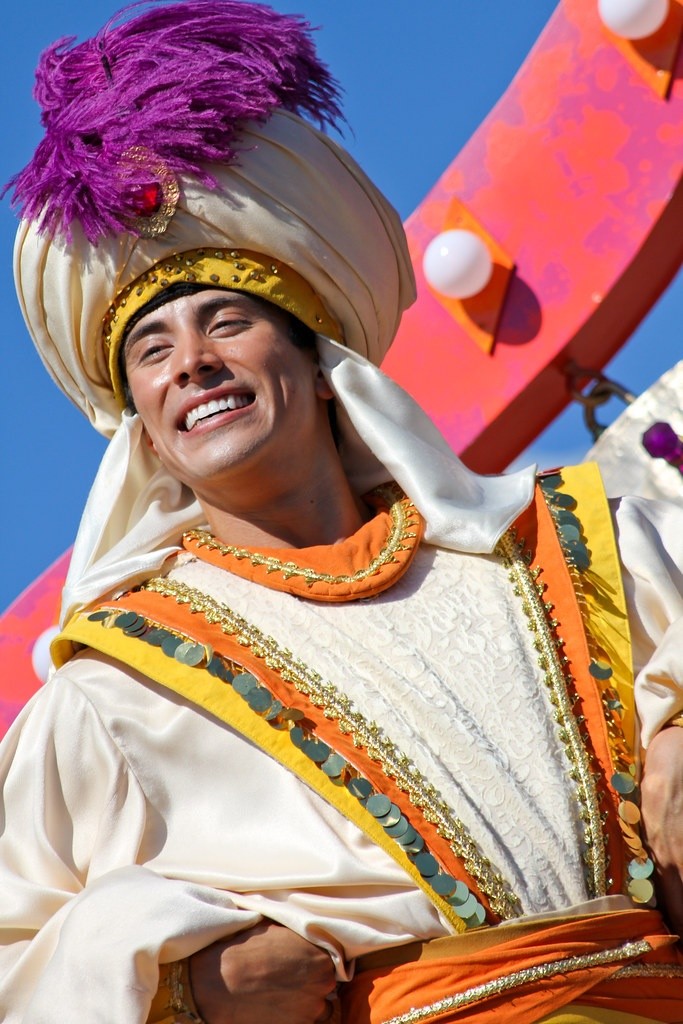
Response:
[12,1,421,436]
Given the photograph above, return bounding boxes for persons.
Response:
[0,97,683,1024]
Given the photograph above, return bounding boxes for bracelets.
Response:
[146,954,205,1024]
